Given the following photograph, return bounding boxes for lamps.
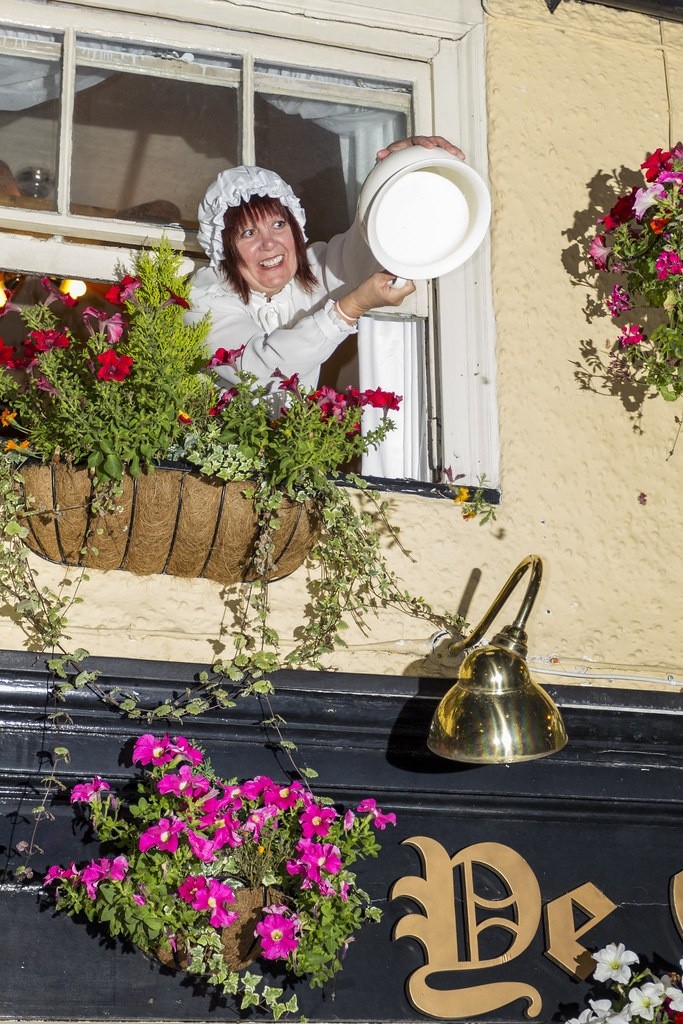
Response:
[427,555,569,764]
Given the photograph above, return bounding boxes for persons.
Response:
[179,136,466,422]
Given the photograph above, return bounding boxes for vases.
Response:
[11,455,327,583]
[155,885,282,974]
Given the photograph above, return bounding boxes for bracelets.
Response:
[333,299,360,322]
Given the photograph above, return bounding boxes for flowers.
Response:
[582,138,683,399]
[2,224,498,1024]
[564,942,683,1024]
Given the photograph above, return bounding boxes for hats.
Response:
[196,165,309,278]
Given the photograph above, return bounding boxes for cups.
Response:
[355,145,492,289]
